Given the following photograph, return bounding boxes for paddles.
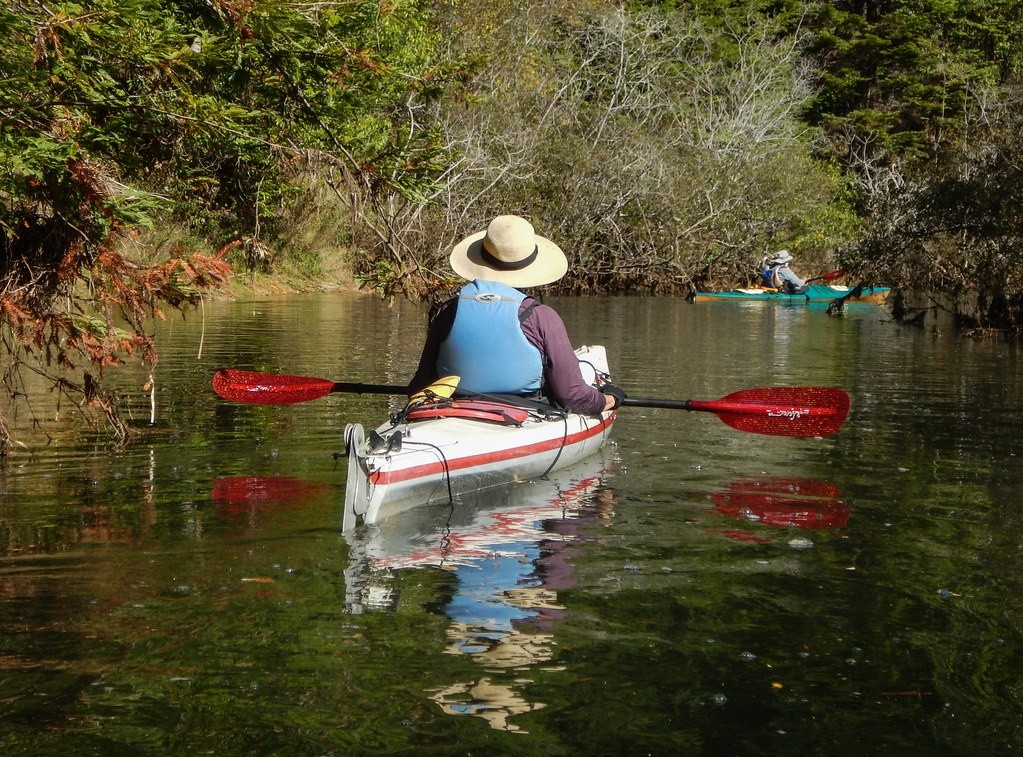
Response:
[805,269,848,285]
[212,367,852,436]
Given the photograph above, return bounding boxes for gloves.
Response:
[600,385,628,411]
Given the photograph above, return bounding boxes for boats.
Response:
[689,285,891,308]
[338,344,622,528]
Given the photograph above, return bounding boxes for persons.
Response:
[764,250,809,294]
[408,214,628,415]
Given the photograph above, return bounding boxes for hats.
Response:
[450,214,568,288]
[771,250,793,263]
